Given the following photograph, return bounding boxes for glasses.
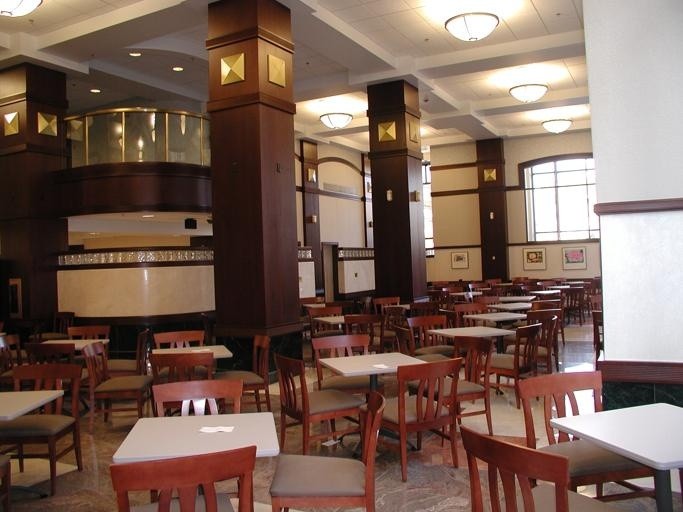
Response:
[509,84,548,104]
[444,12,500,43]
[541,119,572,134]
[320,113,353,130]
[0,0,43,18]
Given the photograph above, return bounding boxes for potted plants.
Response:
[450,251,469,269]
[562,247,587,270]
[523,247,546,270]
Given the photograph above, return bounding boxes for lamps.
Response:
[482,323,543,410]
[67,326,110,340]
[308,306,342,359]
[373,297,400,319]
[0,364,82,495]
[0,334,23,373]
[561,288,583,325]
[427,291,449,309]
[326,302,352,314]
[512,277,528,284]
[513,311,559,361]
[409,336,495,447]
[380,306,407,354]
[272,353,366,456]
[410,301,440,317]
[454,303,486,327]
[359,357,463,482]
[81,341,154,434]
[391,324,448,362]
[592,311,603,371]
[136,328,149,376]
[573,286,591,322]
[442,287,463,293]
[311,334,369,424]
[504,315,558,373]
[557,295,565,346]
[485,279,501,288]
[476,297,499,312]
[148,352,214,385]
[269,390,386,512]
[153,331,204,348]
[152,378,243,417]
[406,315,455,357]
[537,281,557,290]
[108,445,258,512]
[25,342,75,365]
[468,283,487,292]
[215,335,271,414]
[0,454,12,512]
[521,285,543,295]
[517,370,656,501]
[344,314,374,351]
[459,424,569,512]
